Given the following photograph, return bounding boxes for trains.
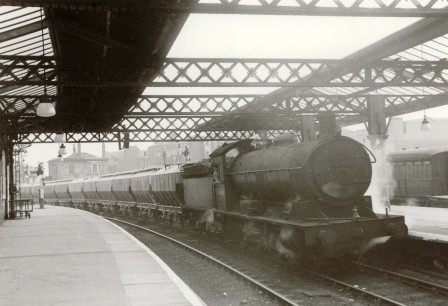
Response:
[23,114,408,272]
[379,147,448,206]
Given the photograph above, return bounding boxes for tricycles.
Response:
[9,187,33,219]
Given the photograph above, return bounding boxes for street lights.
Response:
[14,144,31,217]
